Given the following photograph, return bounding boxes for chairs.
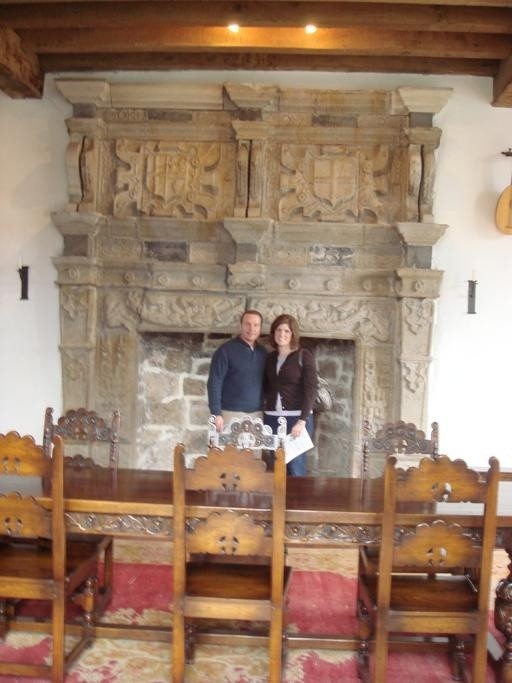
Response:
[172,443,291,682]
[360,420,439,578]
[354,454,500,682]
[207,413,286,462]
[0,430,95,682]
[40,407,121,622]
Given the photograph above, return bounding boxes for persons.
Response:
[263,314,318,478]
[207,311,269,433]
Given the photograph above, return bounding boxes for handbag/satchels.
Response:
[312,377,334,411]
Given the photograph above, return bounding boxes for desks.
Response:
[1,465,511,682]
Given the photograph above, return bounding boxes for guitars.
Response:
[495,150,511,235]
